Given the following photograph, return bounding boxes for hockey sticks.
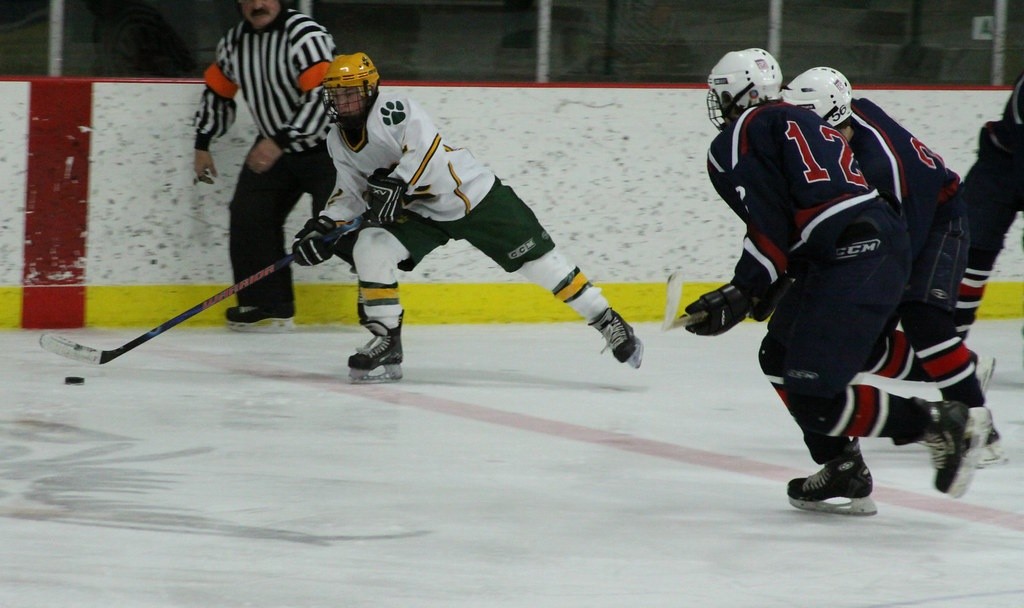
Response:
[661,267,715,335]
[38,206,378,367]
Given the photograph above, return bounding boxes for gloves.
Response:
[681,284,752,336]
[292,214,336,266]
[750,273,793,322]
[362,175,410,228]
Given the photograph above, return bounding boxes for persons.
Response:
[680,47,1024,517]
[292,52,643,385]
[193,0,335,335]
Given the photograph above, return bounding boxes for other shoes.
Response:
[977,426,1008,469]
[969,348,996,395]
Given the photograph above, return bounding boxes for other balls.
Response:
[64,376,85,385]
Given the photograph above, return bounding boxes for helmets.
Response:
[785,66,853,128]
[321,52,380,129]
[707,48,784,133]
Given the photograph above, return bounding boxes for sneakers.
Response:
[894,397,993,498]
[226,305,297,332]
[588,307,644,369]
[347,309,405,385]
[786,437,878,515]
